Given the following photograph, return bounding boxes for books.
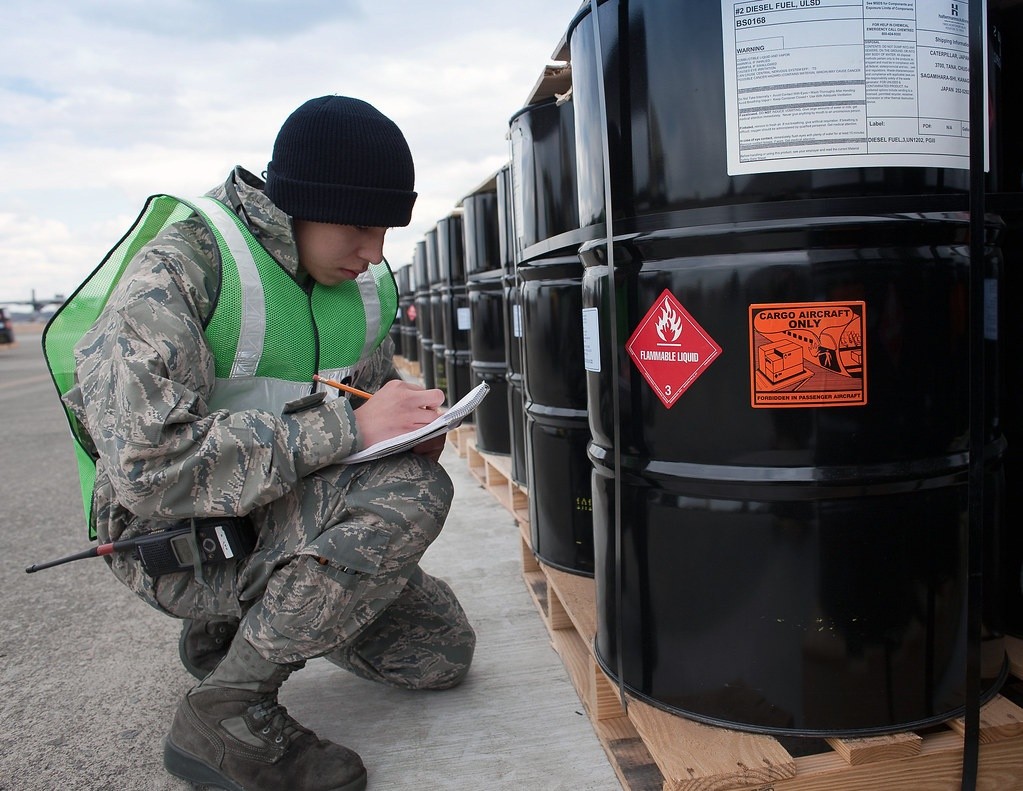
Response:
[336,380,490,464]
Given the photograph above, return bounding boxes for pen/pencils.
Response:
[312,374,374,399]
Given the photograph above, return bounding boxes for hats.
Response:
[265,94,418,227]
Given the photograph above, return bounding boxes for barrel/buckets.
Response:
[391,1,1023,738]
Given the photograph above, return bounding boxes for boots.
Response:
[163,629,367,791]
[179,616,244,679]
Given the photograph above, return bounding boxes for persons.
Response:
[41,95,477,791]
